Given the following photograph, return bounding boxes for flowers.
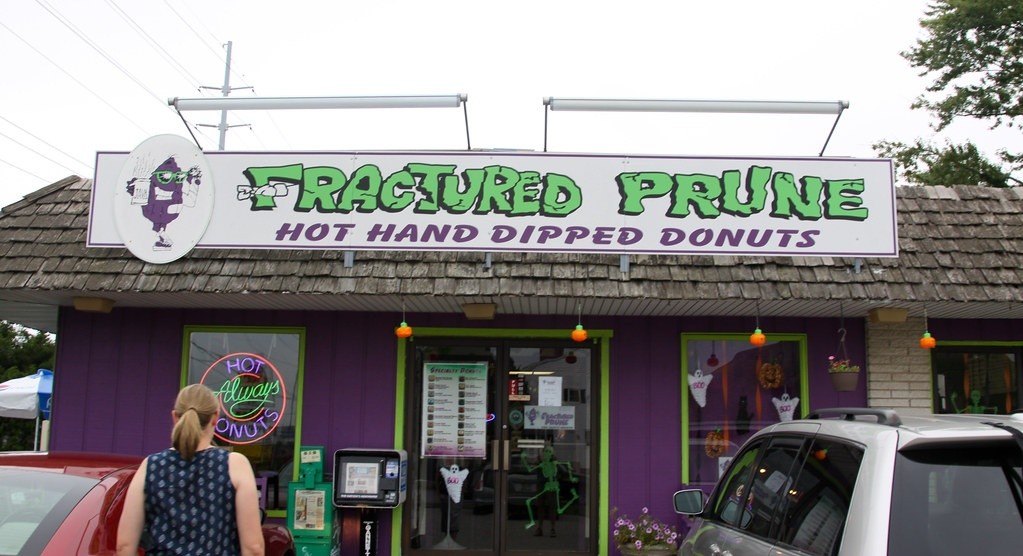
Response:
[828,355,861,373]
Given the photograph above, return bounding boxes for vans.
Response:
[671,406,1017,556]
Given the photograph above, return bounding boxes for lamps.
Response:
[543,96,850,153]
[750,300,766,346]
[572,300,587,342]
[920,307,936,349]
[167,94,470,152]
[395,296,412,338]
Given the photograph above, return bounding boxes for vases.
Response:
[831,372,859,391]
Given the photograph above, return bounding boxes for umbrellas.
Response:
[0,369,53,451]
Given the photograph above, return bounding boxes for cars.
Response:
[2,448,299,553]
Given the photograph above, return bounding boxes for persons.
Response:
[534,431,576,537]
[436,457,464,539]
[117,384,264,556]
